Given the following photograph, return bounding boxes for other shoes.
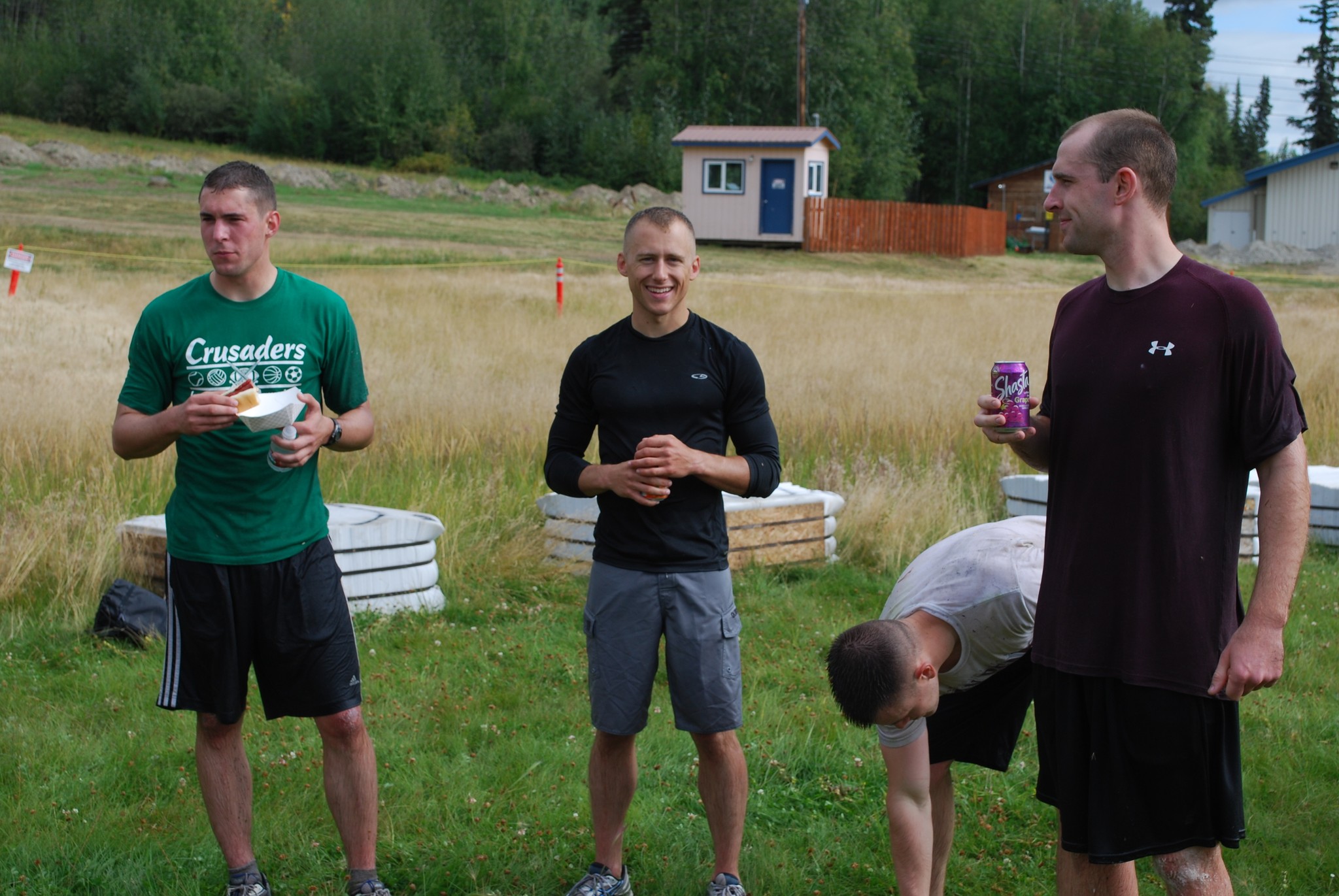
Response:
[227,871,272,896]
[350,881,392,896]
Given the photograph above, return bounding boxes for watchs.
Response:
[320,418,342,448]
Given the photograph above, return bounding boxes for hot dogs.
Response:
[224,380,260,413]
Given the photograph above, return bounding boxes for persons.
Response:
[543,207,783,895]
[825,514,1091,896]
[975,107,1310,896]
[112,159,399,896]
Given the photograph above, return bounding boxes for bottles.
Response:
[266,425,297,472]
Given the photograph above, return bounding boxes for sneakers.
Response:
[566,860,633,896]
[707,872,746,896]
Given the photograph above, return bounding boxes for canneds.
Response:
[641,476,668,500]
[991,361,1030,433]
